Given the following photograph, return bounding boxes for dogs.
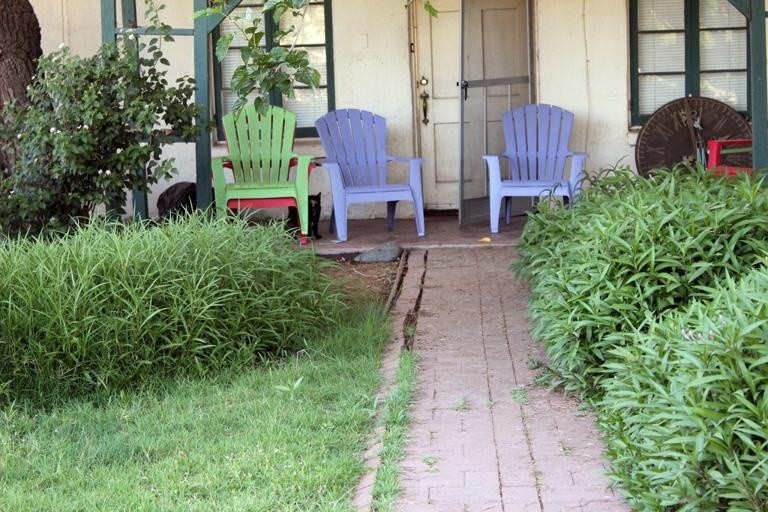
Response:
[156,181,239,223]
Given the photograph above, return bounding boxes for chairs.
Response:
[312,108,427,241]
[210,102,319,246]
[481,102,590,234]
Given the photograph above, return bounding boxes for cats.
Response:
[287,192,322,240]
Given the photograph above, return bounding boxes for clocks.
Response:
[634,96,754,182]
